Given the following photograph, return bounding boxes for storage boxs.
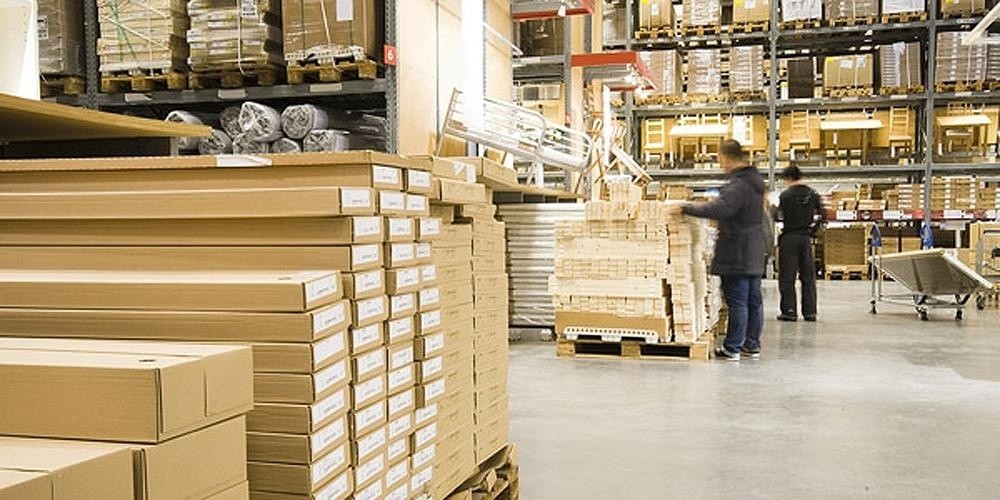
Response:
[0,148,515,500]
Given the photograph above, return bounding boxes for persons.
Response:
[670,139,771,361]
[771,166,825,321]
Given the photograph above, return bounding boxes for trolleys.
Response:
[866,217,994,322]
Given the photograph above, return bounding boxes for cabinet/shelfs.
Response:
[627,3,772,278]
[928,0,1000,280]
[772,0,932,278]
[38,3,624,204]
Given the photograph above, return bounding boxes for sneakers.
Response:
[804,313,816,321]
[777,313,798,321]
[710,346,761,361]
